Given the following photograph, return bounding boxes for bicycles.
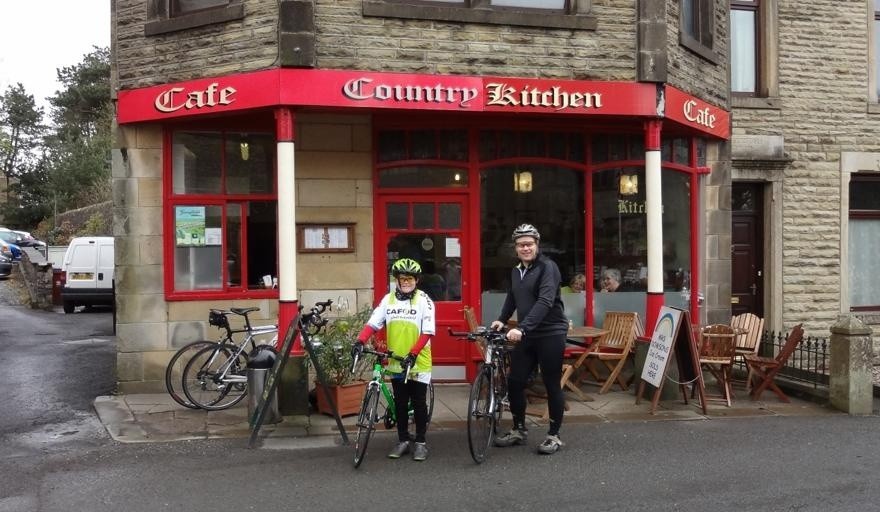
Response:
[446,326,513,465]
[350,346,434,468]
[183,306,329,411]
[163,298,334,409]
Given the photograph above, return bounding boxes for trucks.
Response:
[60,236,114,314]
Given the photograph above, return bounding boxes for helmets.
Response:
[392,258,423,279]
[512,224,540,243]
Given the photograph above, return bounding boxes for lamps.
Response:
[615,166,639,198]
[513,170,533,194]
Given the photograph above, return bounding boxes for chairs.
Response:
[571,311,637,396]
[718,313,764,390]
[743,323,805,404]
[691,324,738,400]
[464,308,485,358]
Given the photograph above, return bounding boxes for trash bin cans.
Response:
[245,344,284,425]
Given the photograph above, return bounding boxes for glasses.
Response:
[397,276,417,282]
[515,242,538,247]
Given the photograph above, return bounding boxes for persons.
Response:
[490,223,570,455]
[600,268,631,293]
[351,258,437,461]
[560,273,587,294]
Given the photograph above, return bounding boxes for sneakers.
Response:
[387,441,411,459]
[412,442,431,461]
[536,435,563,455]
[494,430,528,448]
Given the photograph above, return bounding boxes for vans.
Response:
[0,236,11,261]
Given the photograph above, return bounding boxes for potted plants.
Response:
[303,306,376,417]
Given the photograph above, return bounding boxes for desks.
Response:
[560,326,610,402]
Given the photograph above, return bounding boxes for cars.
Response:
[8,231,47,246]
[0,250,12,278]
[9,243,21,258]
